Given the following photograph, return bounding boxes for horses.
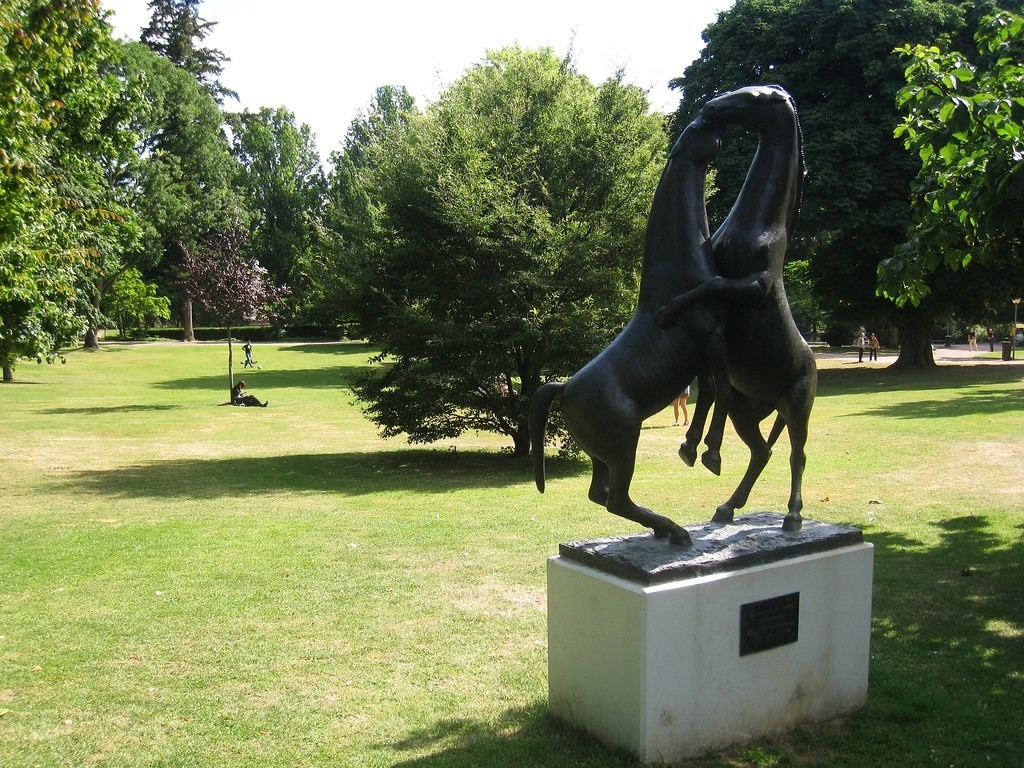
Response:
[527,84,818,550]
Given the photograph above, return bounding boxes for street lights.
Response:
[1012,293,1021,361]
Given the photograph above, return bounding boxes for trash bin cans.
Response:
[1001,339,1012,362]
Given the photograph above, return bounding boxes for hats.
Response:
[870,333,875,336]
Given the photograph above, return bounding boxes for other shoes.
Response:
[683,422,690,425]
[673,424,680,426]
[264,400,268,407]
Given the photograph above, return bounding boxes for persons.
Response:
[857,333,878,362]
[672,385,690,426]
[232,381,269,407]
[968,327,978,351]
[488,384,517,421]
[988,329,994,352]
[1012,332,1024,346]
[242,340,254,368]
